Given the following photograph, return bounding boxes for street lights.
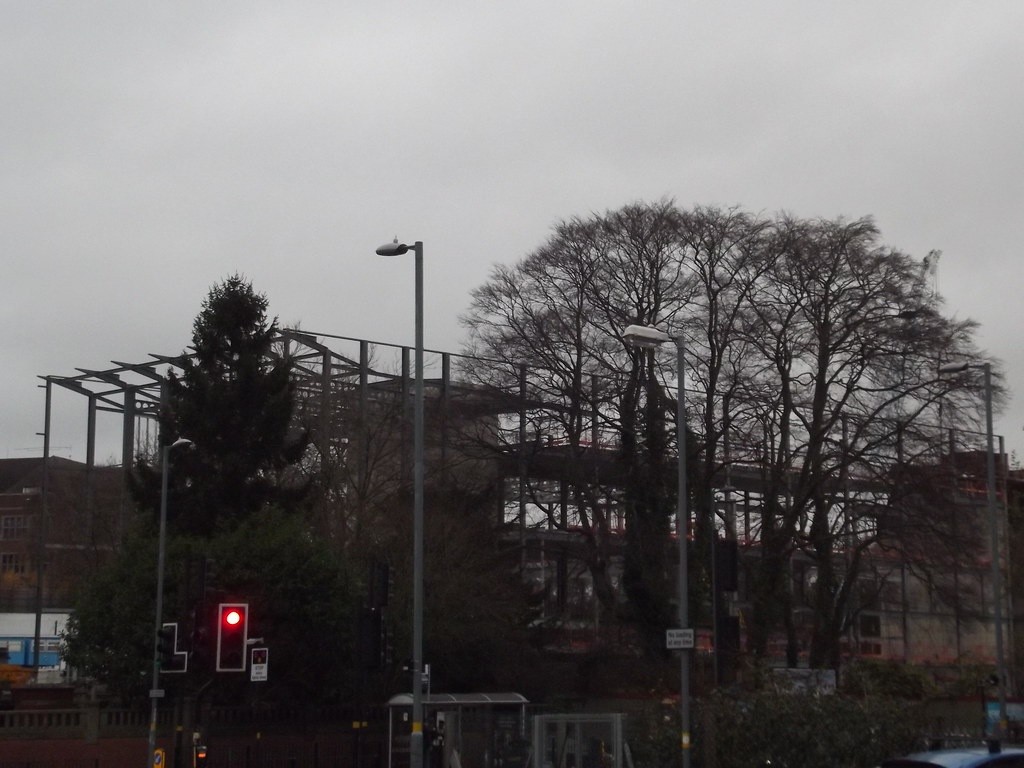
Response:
[146,437,192,768]
[376,238,430,768]
[620,322,699,768]
[943,357,1007,724]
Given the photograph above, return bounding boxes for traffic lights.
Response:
[171,651,187,673]
[215,603,249,671]
[157,620,177,673]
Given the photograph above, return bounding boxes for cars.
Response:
[876,738,1024,768]
